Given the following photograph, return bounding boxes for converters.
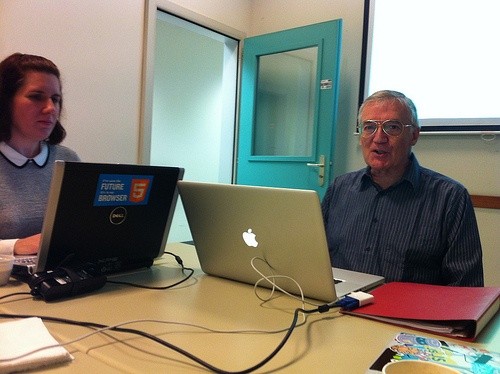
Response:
[28,264,107,301]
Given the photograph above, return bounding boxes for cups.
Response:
[0,254,15,286]
[381,359,462,374]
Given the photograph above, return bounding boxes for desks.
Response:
[0,244,500,374]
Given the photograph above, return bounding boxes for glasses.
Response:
[356,120,415,137]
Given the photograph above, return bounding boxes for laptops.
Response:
[177,180,384,304]
[10,160,185,284]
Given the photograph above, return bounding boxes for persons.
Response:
[0,52,82,256]
[319,90,484,288]
